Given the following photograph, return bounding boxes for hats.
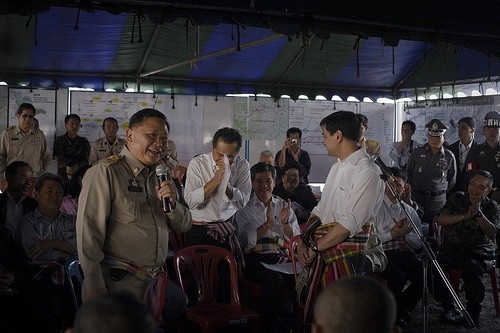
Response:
[481,110,500,129]
[424,118,450,138]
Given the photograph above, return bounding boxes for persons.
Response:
[275,127,311,183]
[389,120,422,167]
[236,162,301,333]
[52,114,91,180]
[261,151,275,166]
[273,163,318,225]
[408,118,457,238]
[183,128,252,303]
[430,169,500,328]
[75,108,192,306]
[60,165,93,215]
[89,117,126,166]
[356,114,380,155]
[375,166,423,326]
[21,172,79,267]
[66,290,151,333]
[447,117,479,191]
[0,161,39,290]
[297,111,385,294]
[311,276,402,333]
[462,111,500,205]
[0,103,51,193]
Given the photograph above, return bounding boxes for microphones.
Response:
[372,153,396,182]
[156,164,171,214]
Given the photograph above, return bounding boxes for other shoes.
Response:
[437,307,459,325]
[461,312,480,329]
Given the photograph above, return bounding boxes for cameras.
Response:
[292,139,297,144]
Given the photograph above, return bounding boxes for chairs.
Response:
[30,220,307,333]
[419,211,500,333]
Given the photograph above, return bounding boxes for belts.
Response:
[413,189,446,197]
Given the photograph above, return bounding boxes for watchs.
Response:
[474,211,481,217]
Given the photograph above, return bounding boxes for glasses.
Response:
[283,171,300,178]
